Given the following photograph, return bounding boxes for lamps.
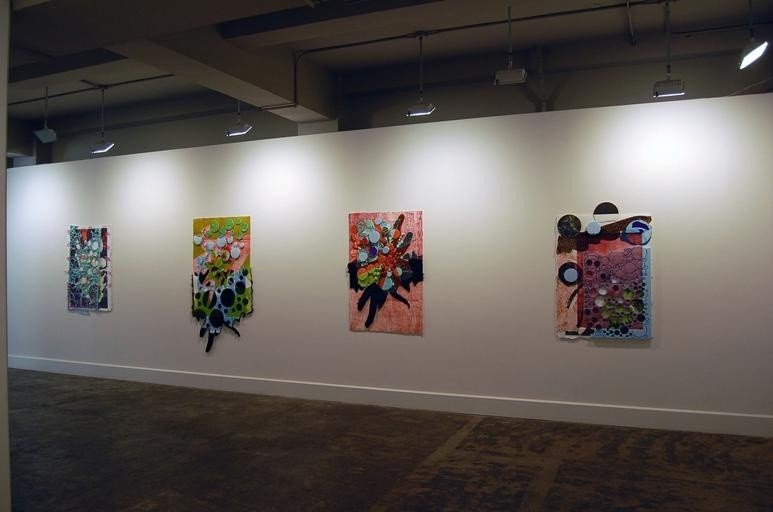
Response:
[738,40,768,73]
[86,132,118,155]
[403,99,438,120]
[33,122,60,145]
[224,119,254,141]
[650,76,688,100]
[493,67,528,84]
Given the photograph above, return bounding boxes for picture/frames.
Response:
[65,224,114,312]
